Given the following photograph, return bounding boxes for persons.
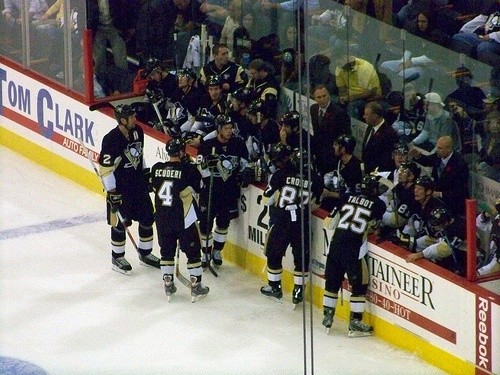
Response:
[98,103,162,274]
[374,139,416,195]
[373,162,443,242]
[263,111,321,177]
[411,134,472,224]
[405,207,484,274]
[259,143,314,310]
[318,136,371,215]
[359,102,399,189]
[0,0,500,159]
[150,138,209,302]
[475,198,500,277]
[310,84,354,173]
[322,179,386,337]
[196,114,250,267]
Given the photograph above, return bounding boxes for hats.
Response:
[424,93,445,105]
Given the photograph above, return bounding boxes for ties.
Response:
[318,109,324,127]
[370,128,375,140]
[438,162,445,176]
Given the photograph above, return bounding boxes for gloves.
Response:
[236,164,253,189]
[107,191,124,213]
[203,175,220,193]
[144,173,154,192]
[390,229,416,252]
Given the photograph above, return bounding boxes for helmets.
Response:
[115,104,136,118]
[144,59,161,78]
[208,75,223,87]
[426,206,453,225]
[335,135,356,146]
[392,144,436,190]
[178,68,197,81]
[362,176,381,190]
[166,138,187,157]
[273,109,314,170]
[216,88,269,121]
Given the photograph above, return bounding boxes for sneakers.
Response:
[322,309,335,333]
[349,319,374,337]
[293,284,308,309]
[163,273,176,300]
[201,249,223,272]
[112,256,132,276]
[139,252,161,270]
[190,274,208,303]
[260,285,282,303]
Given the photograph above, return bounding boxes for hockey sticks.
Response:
[206,147,218,278]
[78,138,137,250]
[90,101,117,112]
[176,243,192,290]
